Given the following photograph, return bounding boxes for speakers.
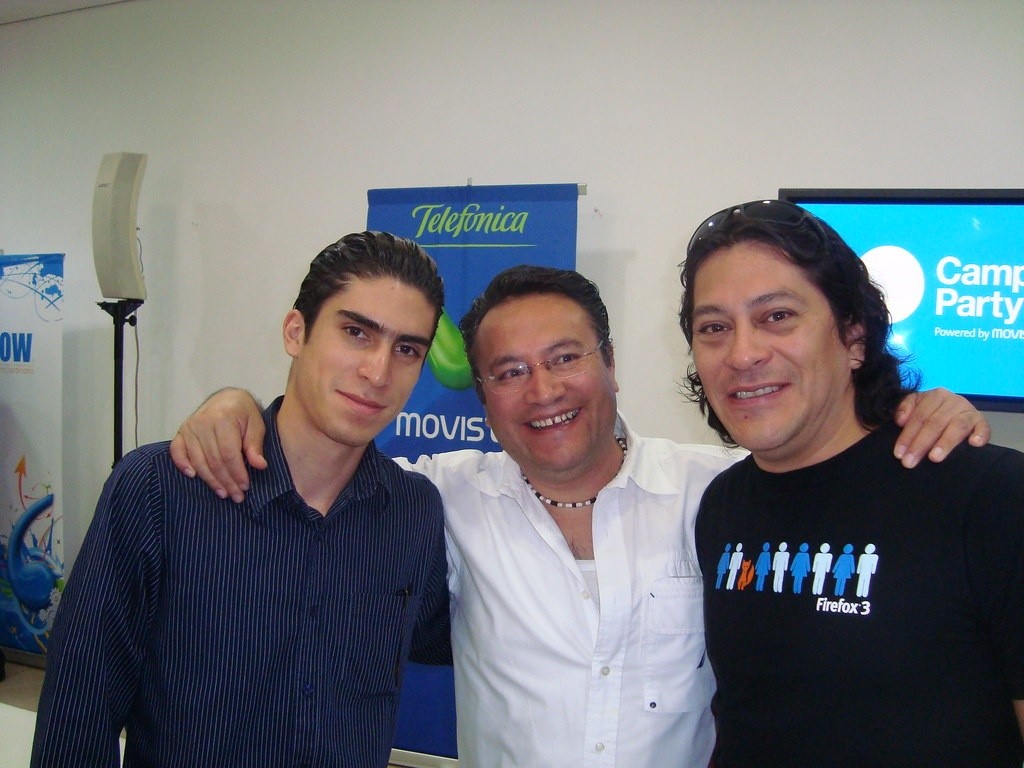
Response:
[93,151,147,301]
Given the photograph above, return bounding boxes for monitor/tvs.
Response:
[776,188,1024,414]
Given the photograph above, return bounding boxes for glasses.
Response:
[688,199,828,252]
[475,341,604,395]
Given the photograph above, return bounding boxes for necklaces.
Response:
[519,434,629,509]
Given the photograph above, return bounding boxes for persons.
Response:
[167,263,989,768]
[29,229,456,768]
[674,198,1024,768]
